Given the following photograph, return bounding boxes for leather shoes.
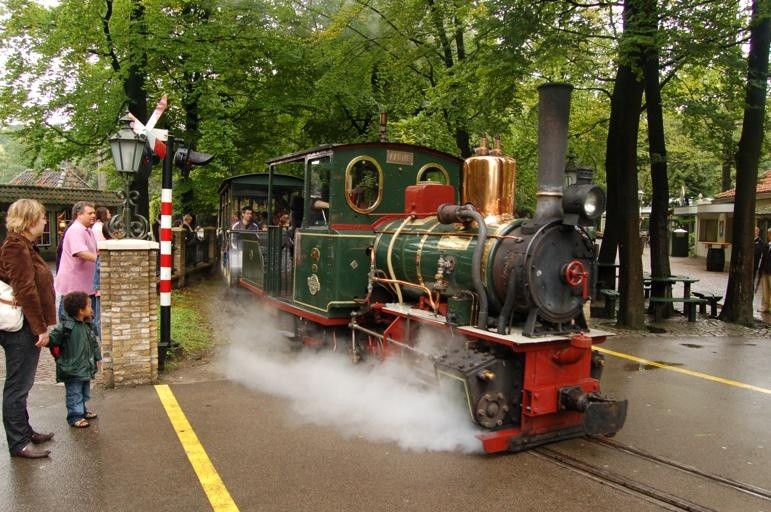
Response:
[32,431,53,443]
[19,443,50,458]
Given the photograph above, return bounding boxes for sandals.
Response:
[74,419,89,428]
[85,410,97,418]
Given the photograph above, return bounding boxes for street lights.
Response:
[102,108,153,242]
[564,144,578,189]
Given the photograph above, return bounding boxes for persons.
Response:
[180,214,194,232]
[231,207,291,230]
[0,196,115,459]
[757,227,771,313]
[755,227,762,288]
[310,196,330,209]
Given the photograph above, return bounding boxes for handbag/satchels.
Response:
[0,280,24,333]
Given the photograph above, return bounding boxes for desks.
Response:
[641,274,699,314]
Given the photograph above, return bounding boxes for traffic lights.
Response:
[186,149,216,170]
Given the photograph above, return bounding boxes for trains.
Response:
[217,82,628,457]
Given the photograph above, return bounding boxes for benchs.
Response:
[599,288,620,318]
[692,291,723,316]
[648,296,706,322]
[643,287,651,298]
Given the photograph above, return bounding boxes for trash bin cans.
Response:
[671,228,688,257]
[702,242,730,272]
